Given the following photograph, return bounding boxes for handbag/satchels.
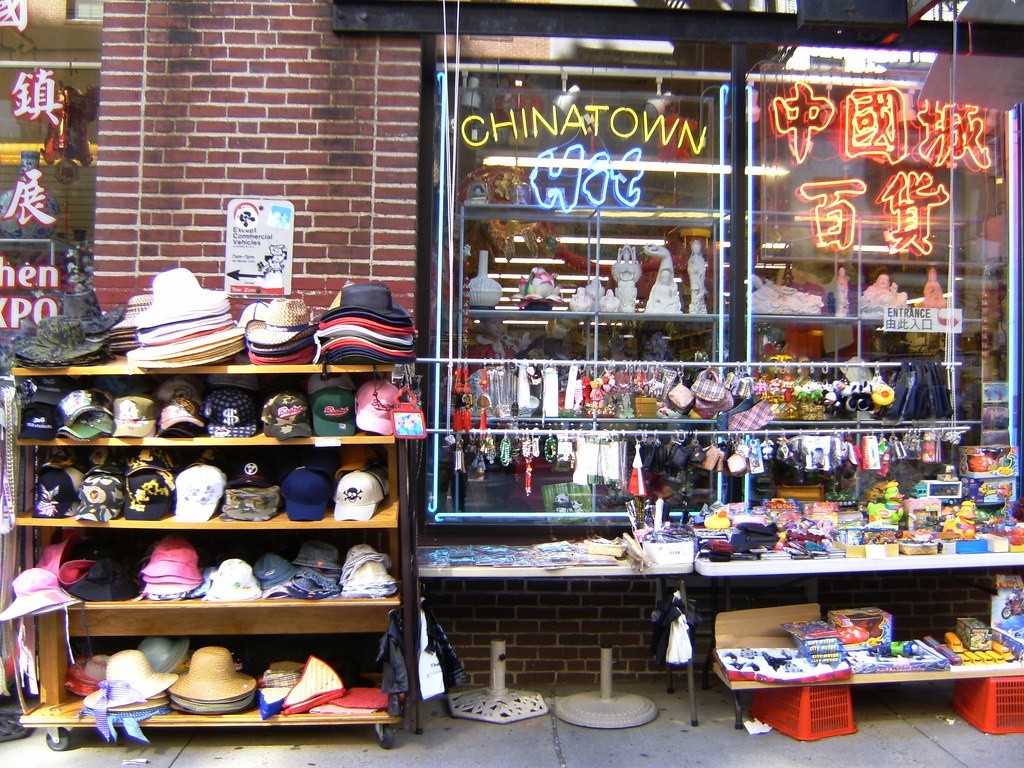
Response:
[878,360,954,421]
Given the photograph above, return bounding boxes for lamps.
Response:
[460,77,481,110]
[746,90,761,123]
[644,91,674,119]
[476,148,791,177]
[552,85,581,118]
[818,98,839,127]
[897,93,917,129]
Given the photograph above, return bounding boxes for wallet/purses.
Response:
[639,366,773,478]
[698,540,733,562]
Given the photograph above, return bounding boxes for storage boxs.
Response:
[958,442,1020,479]
[791,634,842,666]
[712,603,851,684]
[844,639,951,676]
[950,676,1024,735]
[990,574,1024,660]
[961,477,1016,504]
[748,684,859,741]
[642,540,694,565]
[956,616,992,651]
[827,607,892,649]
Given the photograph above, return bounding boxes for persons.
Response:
[466,316,520,375]
[611,240,948,320]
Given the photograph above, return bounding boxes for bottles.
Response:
[467,251,503,310]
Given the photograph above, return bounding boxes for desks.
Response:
[694,523,1024,731]
[412,546,699,735]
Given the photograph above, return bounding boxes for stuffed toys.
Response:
[821,377,894,415]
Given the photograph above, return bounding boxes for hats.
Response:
[0,266,425,743]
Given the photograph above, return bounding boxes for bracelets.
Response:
[486,435,555,466]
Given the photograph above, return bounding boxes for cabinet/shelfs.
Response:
[11,353,402,753]
[454,200,988,512]
